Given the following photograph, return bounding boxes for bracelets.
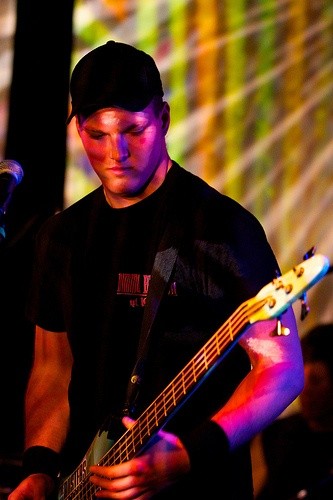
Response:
[176,417,233,472]
[24,446,61,480]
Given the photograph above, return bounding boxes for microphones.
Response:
[0,160,24,216]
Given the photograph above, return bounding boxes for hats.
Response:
[67,40,164,125]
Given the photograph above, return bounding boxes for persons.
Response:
[7,40,304,500]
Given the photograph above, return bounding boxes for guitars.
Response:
[43,245,333,500]
